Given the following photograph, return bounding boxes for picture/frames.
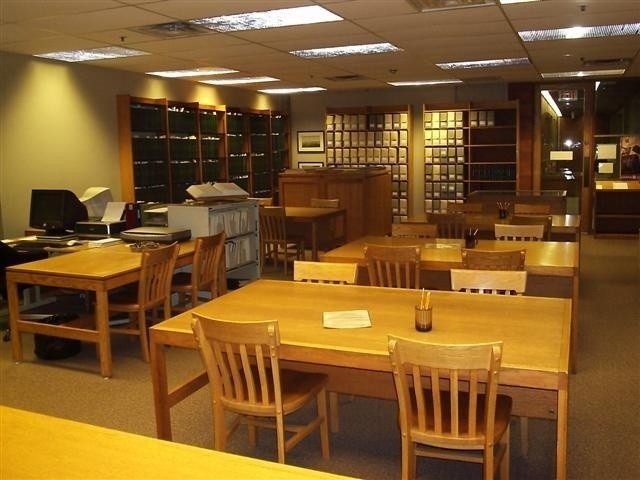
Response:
[298,162,324,170]
[297,131,326,154]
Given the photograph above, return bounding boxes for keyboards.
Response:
[14,241,67,248]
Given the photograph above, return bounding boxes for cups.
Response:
[415,304,432,332]
[464,235,479,249]
[498,209,509,220]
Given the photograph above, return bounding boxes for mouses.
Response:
[67,239,82,247]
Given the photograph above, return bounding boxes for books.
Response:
[87,237,122,248]
[108,313,131,327]
[185,179,257,272]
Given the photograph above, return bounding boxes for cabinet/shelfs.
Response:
[116,93,291,205]
[592,181,640,240]
[462,97,520,203]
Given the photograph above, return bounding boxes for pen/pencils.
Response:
[496,200,511,210]
[469,228,472,237]
[473,228,479,237]
[420,288,431,310]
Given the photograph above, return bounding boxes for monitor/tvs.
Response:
[30,189,88,236]
[78,188,115,223]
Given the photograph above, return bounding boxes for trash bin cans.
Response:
[32,315,82,360]
